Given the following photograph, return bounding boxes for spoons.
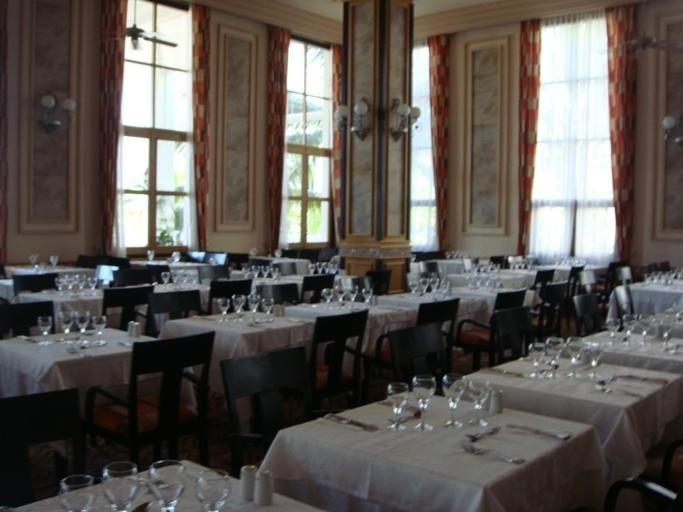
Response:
[131,500,151,511]
[462,442,524,464]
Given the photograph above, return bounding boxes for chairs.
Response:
[0,246,682,511]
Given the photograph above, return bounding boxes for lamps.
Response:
[38,94,77,134]
[334,97,421,143]
[661,114,682,147]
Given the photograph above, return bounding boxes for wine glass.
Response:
[468,378,488,425]
[305,247,586,307]
[410,374,435,433]
[25,246,282,348]
[528,267,683,382]
[386,381,408,434]
[442,372,465,430]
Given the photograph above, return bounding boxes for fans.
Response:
[97,0,177,49]
[630,0,683,51]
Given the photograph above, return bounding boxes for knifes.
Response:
[324,412,377,431]
[489,366,523,377]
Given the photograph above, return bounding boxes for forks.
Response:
[594,385,643,399]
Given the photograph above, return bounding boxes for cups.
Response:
[58,473,94,511]
[147,459,184,510]
[100,460,137,511]
[193,468,231,511]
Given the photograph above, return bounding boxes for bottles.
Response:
[239,464,258,502]
[256,467,274,506]
[480,387,503,414]
